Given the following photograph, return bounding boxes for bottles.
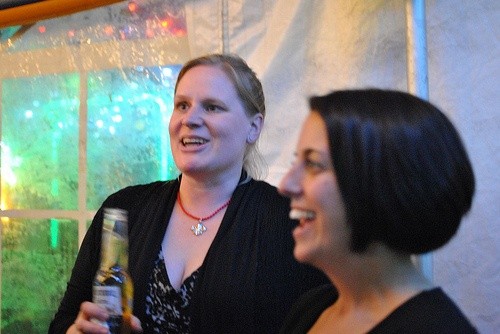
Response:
[92,208,133,334]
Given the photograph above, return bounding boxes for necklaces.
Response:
[176,191,232,235]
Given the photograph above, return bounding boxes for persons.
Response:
[277,89,477,334]
[48,55,323,334]
